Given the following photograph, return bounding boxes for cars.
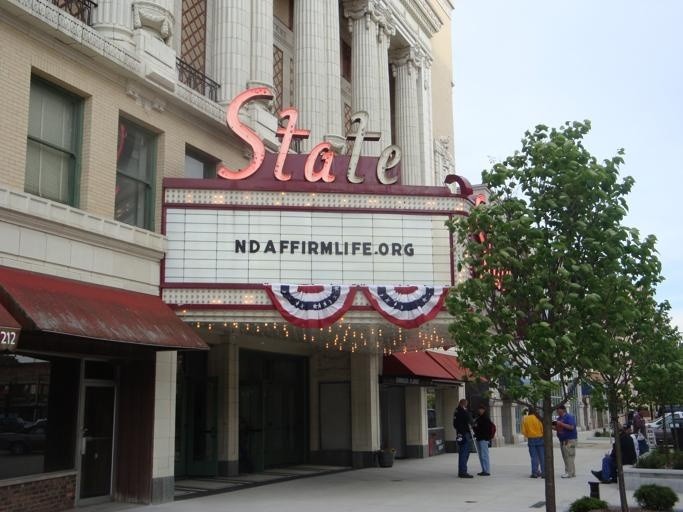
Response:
[645,405,683,445]
[0,412,46,456]
[427,408,436,428]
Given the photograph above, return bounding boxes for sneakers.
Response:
[560,472,577,478]
[540,475,545,479]
[528,474,537,478]
[476,471,490,476]
[457,473,473,478]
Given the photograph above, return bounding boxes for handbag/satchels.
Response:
[455,435,467,447]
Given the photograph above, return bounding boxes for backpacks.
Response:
[488,419,497,439]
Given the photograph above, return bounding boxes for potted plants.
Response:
[378,449,396,467]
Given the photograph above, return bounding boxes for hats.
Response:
[622,424,630,429]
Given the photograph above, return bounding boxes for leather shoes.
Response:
[590,469,603,481]
[600,476,618,484]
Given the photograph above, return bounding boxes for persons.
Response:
[522,409,530,441]
[472,404,491,477]
[588,404,648,485]
[528,405,544,476]
[551,405,577,479]
[520,407,545,479]
[452,398,474,479]
